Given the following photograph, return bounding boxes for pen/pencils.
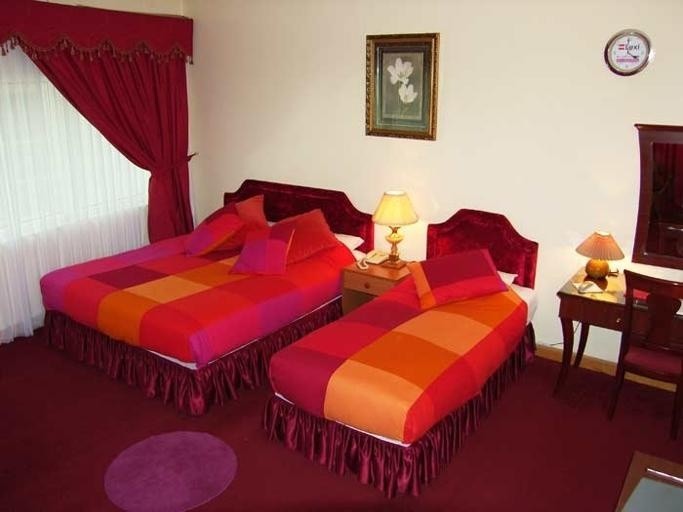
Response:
[581,280,594,291]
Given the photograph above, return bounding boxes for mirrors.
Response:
[630,121,683,272]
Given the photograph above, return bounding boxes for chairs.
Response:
[607,271,683,442]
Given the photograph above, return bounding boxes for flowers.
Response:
[386,57,418,117]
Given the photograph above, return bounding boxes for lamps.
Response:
[371,190,420,270]
[575,230,623,278]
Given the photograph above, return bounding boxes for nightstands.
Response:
[556,266,682,389]
[342,257,413,316]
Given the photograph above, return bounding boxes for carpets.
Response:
[103,431,238,512]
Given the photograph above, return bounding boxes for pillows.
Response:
[214,192,272,252]
[184,202,242,260]
[227,228,294,278]
[274,208,337,265]
[405,248,510,310]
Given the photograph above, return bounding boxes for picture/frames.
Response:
[364,32,439,140]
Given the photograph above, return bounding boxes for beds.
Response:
[263,209,539,498]
[39,179,375,419]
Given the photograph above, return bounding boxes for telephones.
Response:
[356,250,390,270]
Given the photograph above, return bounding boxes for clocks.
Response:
[604,29,654,77]
